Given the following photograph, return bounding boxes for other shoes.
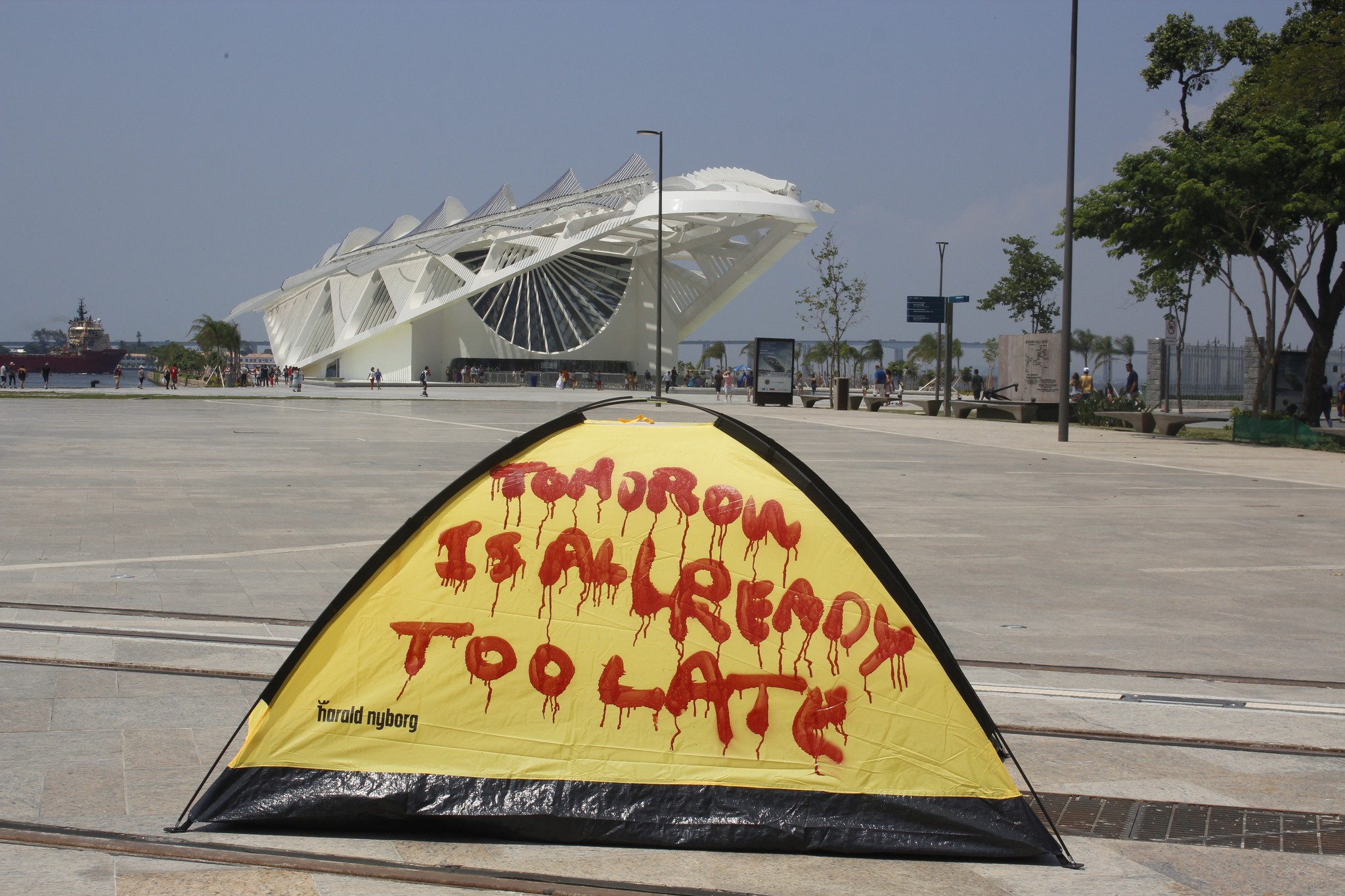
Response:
[140,388,143,389]
[174,388,177,389]
[166,388,169,389]
[116,388,118,389]
[170,385,173,389]
[724,400,728,402]
[138,386,140,388]
[44,386,47,389]
[14,387,18,388]
[1339,420,1345,423]
[3,386,6,388]
[729,400,731,402]
[9,387,12,388]
[20,387,24,389]
[0,384,3,388]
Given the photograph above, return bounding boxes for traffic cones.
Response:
[1161,400,1172,412]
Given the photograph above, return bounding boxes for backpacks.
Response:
[420,370,427,381]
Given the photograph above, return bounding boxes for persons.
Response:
[624,369,652,391]
[713,369,733,403]
[1319,373,1345,429]
[0,361,52,389]
[662,365,713,394]
[860,365,904,407]
[556,367,604,391]
[512,368,526,388]
[1069,363,1140,402]
[114,364,125,389]
[445,363,500,384]
[810,374,817,395]
[137,365,146,389]
[420,365,431,397]
[742,370,754,401]
[796,372,806,397]
[240,363,304,392]
[367,367,383,391]
[968,368,986,400]
[164,363,180,390]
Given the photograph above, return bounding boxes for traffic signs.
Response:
[906,301,945,310]
[907,316,945,323]
[906,295,946,303]
[949,295,966,303]
[906,309,945,316]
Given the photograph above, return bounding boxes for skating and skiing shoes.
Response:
[420,392,428,397]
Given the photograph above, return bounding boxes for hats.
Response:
[139,365,144,368]
[117,364,121,368]
[10,362,15,365]
[1084,367,1089,371]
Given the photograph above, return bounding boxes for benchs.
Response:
[901,399,944,416]
[829,396,863,410]
[1152,413,1230,438]
[800,394,830,407]
[987,404,1038,422]
[862,396,901,412]
[1094,411,1156,433]
[949,400,996,420]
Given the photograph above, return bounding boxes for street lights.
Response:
[935,241,949,401]
[633,129,664,408]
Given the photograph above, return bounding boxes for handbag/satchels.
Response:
[369,377,373,379]
[165,370,170,376]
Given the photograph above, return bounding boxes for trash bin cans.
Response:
[530,375,537,387]
[737,379,742,387]
[1141,383,1147,408]
[456,372,461,382]
[678,376,684,385]
[226,373,236,387]
[834,377,850,410]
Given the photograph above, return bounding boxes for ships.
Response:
[0,296,128,375]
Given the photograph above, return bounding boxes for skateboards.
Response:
[890,401,899,406]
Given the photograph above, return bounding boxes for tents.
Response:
[168,390,1088,871]
